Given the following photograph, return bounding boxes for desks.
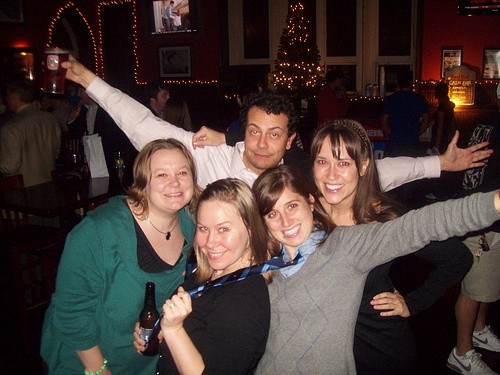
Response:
[345,115,434,142]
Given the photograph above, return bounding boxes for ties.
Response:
[155,246,303,331]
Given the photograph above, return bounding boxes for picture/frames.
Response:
[440,46,464,80]
[157,43,193,79]
[481,47,500,80]
[0,1,27,27]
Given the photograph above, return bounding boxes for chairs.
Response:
[61,136,86,168]
[0,174,67,356]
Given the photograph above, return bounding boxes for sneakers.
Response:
[448,347,498,375]
[472,325,500,352]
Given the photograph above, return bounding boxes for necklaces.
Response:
[148,218,180,241]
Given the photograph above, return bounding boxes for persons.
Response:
[161,0,178,32]
[166,52,189,74]
[253,164,500,375]
[134,177,271,375]
[194,119,474,375]
[446,82,500,375]
[39,138,197,375]
[41,55,493,195]
[0,64,454,206]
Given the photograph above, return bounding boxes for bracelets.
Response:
[84,358,108,375]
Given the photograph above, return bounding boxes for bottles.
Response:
[139,282,160,356]
[116,151,126,168]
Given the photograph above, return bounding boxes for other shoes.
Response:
[443,192,457,199]
[424,193,439,200]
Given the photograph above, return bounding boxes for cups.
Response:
[44,49,71,95]
